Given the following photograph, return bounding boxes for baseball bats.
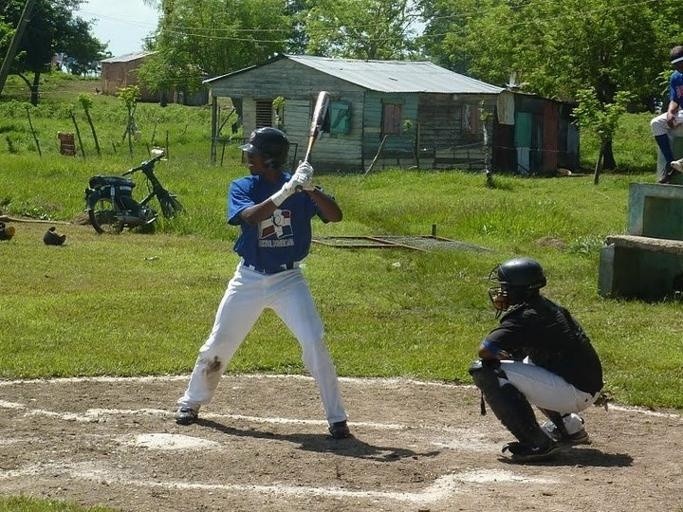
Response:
[294,91,330,193]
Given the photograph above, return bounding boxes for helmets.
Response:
[238,127,289,167]
[488,258,546,311]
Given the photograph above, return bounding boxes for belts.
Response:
[240,260,300,275]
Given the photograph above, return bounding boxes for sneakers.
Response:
[497,441,560,461]
[657,163,676,184]
[175,406,198,425]
[328,419,349,439]
[670,158,683,172]
[539,420,589,444]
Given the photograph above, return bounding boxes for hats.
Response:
[669,46,683,64]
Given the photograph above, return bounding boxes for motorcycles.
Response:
[85,152,183,234]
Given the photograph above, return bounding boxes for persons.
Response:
[649,45,682,186]
[173,126,350,441]
[468,258,604,461]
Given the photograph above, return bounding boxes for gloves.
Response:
[296,160,315,192]
[270,176,306,209]
